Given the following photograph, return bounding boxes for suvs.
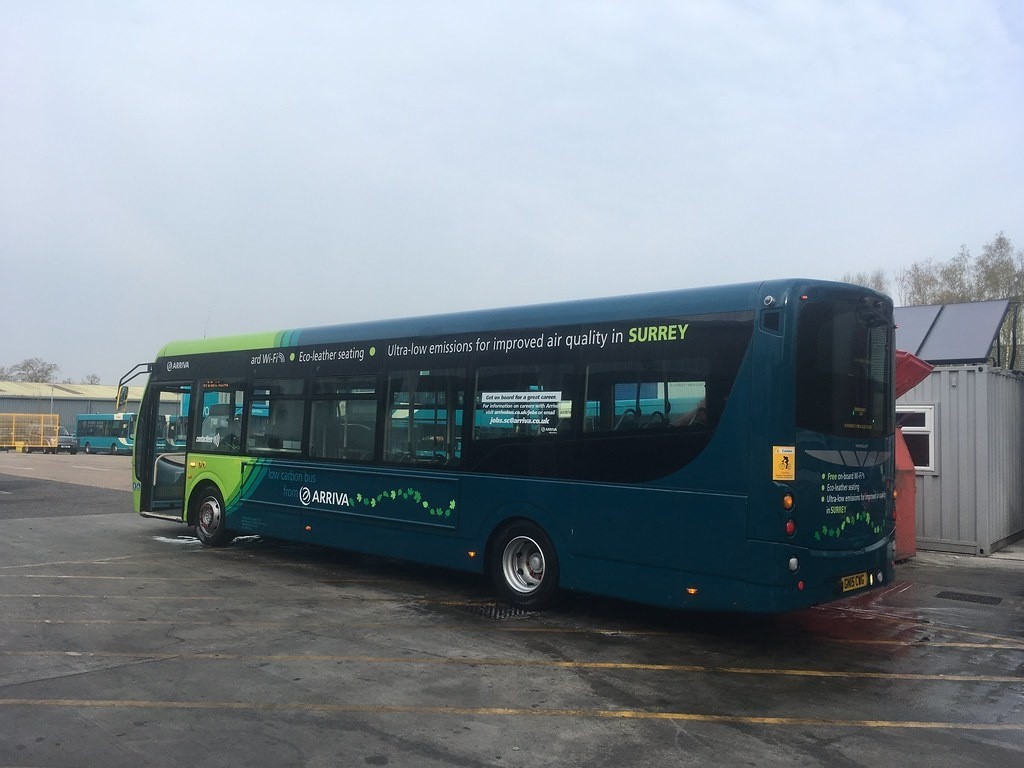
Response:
[23,423,77,455]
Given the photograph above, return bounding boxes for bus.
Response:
[222,375,717,481]
[74,411,180,455]
[166,414,191,450]
[114,276,899,622]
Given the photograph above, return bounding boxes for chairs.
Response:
[204,400,709,478]
